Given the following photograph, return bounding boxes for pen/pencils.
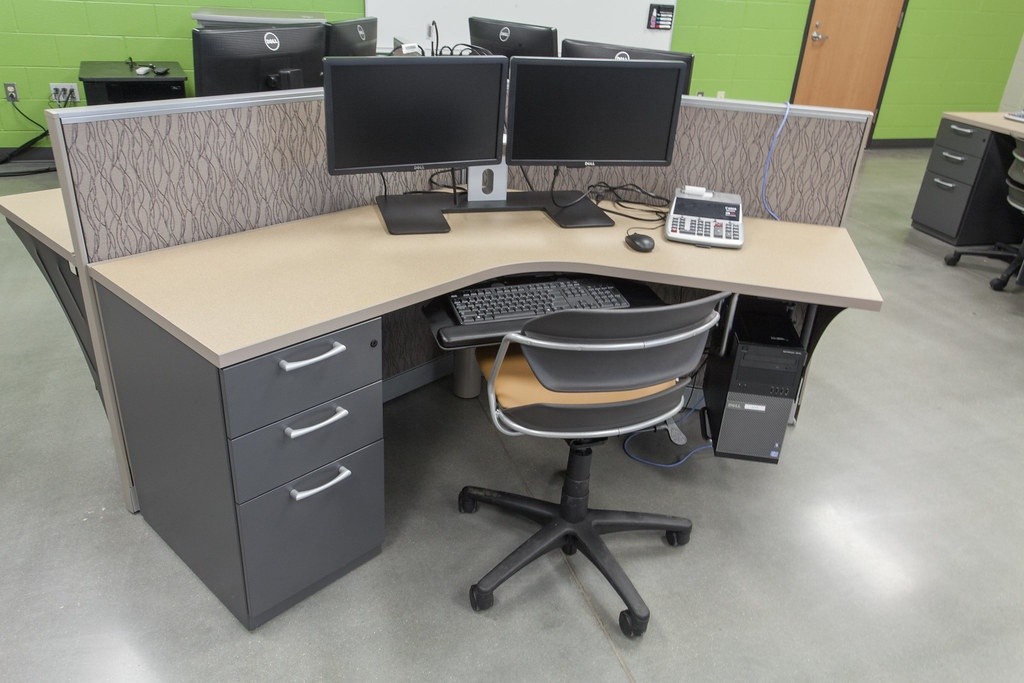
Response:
[139,64,156,69]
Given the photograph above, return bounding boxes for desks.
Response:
[911,112,1023,247]
[78,61,188,105]
[0,84,884,634]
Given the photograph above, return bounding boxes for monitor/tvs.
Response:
[193,16,695,235]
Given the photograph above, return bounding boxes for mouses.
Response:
[625,233,655,252]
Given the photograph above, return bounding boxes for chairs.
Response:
[944,130,1024,292]
[459,289,734,638]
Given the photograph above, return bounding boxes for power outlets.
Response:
[4,83,18,102]
[51,83,80,102]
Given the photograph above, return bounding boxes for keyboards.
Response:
[445,278,630,326]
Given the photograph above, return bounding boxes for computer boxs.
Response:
[702,292,807,464]
